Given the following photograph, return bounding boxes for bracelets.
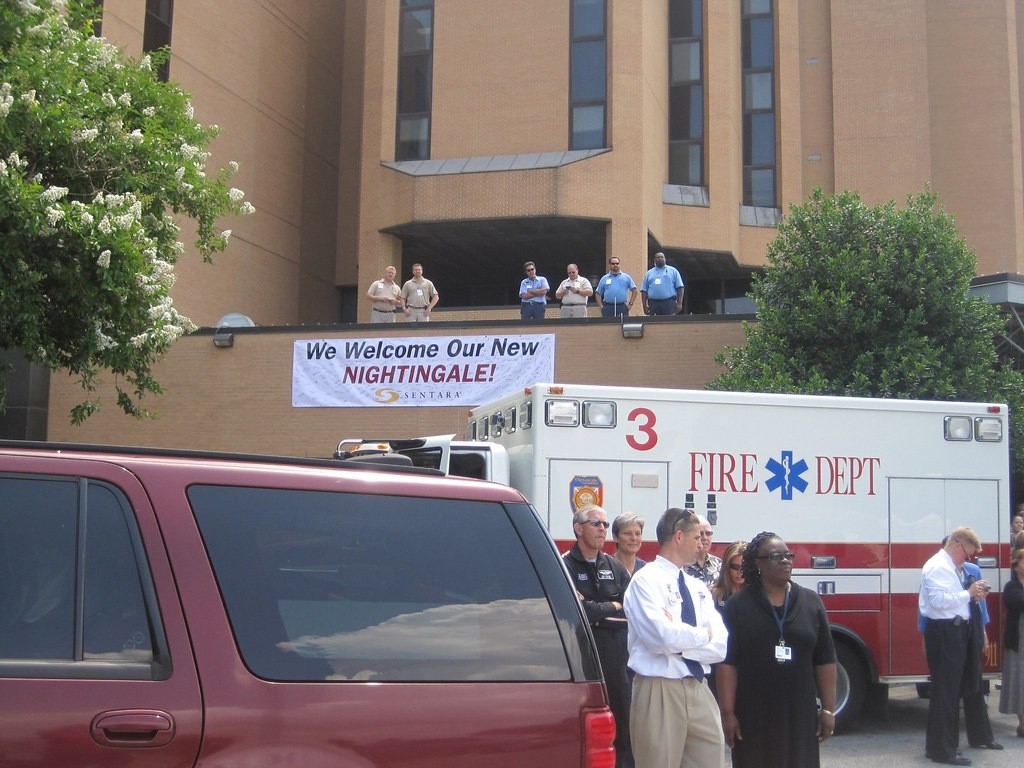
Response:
[822,708,834,716]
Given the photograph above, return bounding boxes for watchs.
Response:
[629,302,633,305]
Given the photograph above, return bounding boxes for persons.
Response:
[716,533,837,768]
[396,263,439,321]
[596,258,637,316]
[366,266,402,323]
[622,507,727,768]
[517,262,550,318]
[560,505,648,768]
[640,253,685,315]
[918,528,1004,765]
[785,649,789,655]
[998,502,1024,740]
[555,264,593,318]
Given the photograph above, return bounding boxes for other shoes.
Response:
[926,748,971,764]
[978,739,1003,749]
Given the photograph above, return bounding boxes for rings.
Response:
[979,587,981,589]
[831,731,834,735]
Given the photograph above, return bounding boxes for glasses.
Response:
[610,263,620,265]
[700,531,713,536]
[526,268,535,272]
[672,507,696,533]
[755,553,795,561]
[729,563,744,571]
[580,519,609,529]
[955,538,972,563]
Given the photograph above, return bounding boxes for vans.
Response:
[0,434,618,764]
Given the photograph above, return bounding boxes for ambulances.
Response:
[318,383,1011,734]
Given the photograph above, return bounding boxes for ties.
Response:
[677,570,704,685]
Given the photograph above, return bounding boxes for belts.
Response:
[373,308,394,312]
[562,303,585,306]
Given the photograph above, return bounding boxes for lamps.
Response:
[213,333,234,347]
[623,323,644,337]
